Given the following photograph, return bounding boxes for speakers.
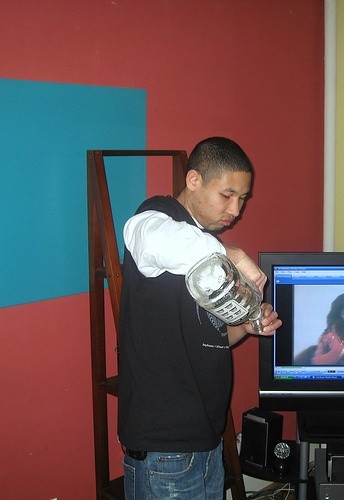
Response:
[240,407,283,467]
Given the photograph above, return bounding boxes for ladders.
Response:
[86,149,246,500]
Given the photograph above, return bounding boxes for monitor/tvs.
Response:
[258,252,344,411]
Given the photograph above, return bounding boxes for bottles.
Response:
[185,252,264,326]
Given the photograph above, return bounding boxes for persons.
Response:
[117,135,284,500]
[313,293,344,365]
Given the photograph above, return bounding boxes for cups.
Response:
[251,319,262,332]
